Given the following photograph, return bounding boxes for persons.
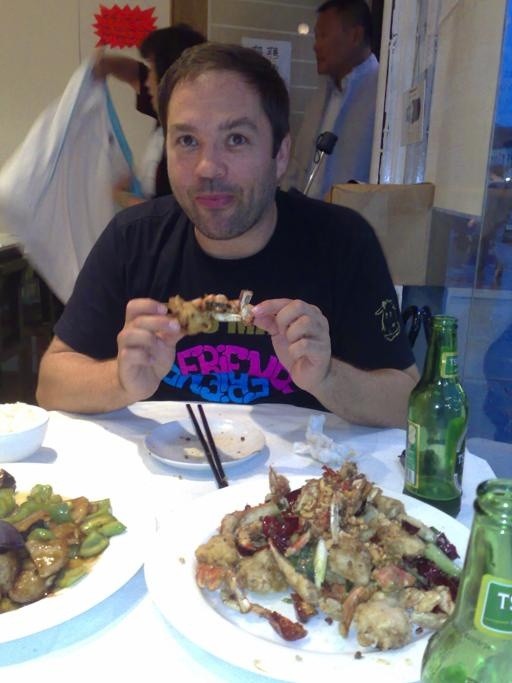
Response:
[280,0,379,204]
[90,23,210,208]
[36,43,421,431]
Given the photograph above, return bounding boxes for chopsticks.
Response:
[184,401,230,487]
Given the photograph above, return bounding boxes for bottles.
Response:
[405,314,470,520]
[422,478,511,682]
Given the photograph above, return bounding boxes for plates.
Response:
[146,419,268,470]
[1,484,155,645]
[142,474,472,683]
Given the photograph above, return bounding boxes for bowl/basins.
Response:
[1,400,51,462]
